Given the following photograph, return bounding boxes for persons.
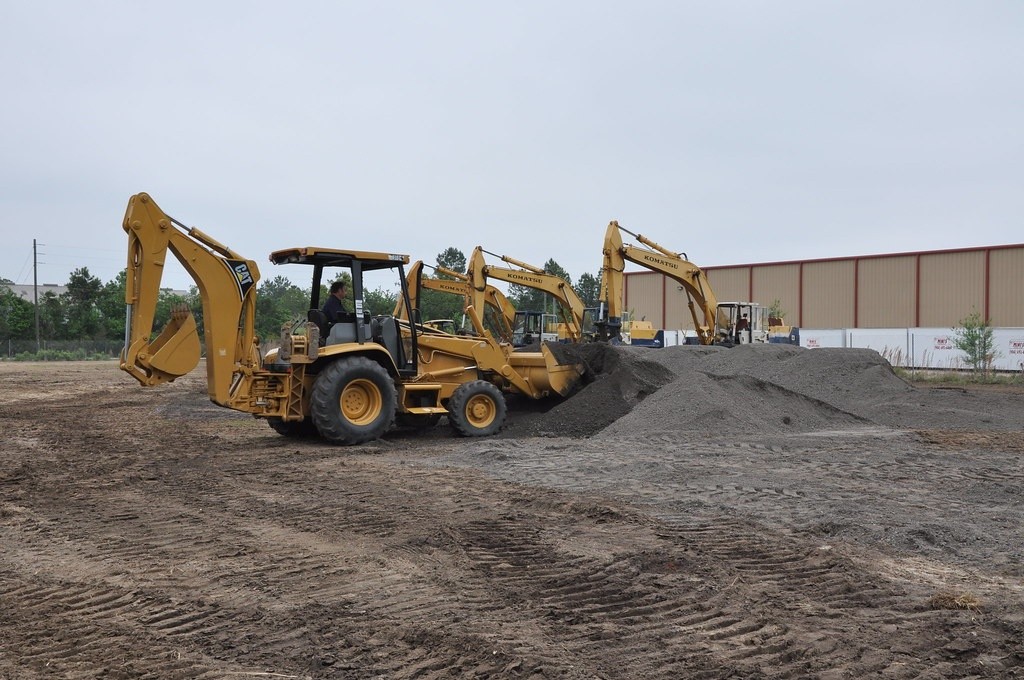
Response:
[321,281,347,322]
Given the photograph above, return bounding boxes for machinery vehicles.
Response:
[119,191,587,448]
[583,220,771,351]
[388,259,560,350]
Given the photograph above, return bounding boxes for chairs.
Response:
[737,319,749,333]
[307,309,333,338]
